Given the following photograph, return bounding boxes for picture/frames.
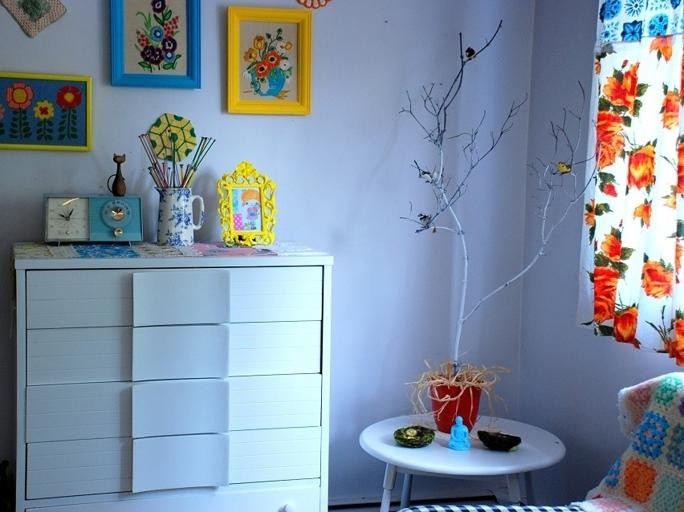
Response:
[109,0,201,88]
[217,161,277,246]
[227,5,312,116]
[0,71,92,152]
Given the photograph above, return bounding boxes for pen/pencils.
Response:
[147,162,197,189]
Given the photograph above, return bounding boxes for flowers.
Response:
[243,27,294,82]
[580,0,684,367]
[133,0,180,72]
[0,82,81,142]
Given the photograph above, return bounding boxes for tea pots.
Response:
[153,183,206,247]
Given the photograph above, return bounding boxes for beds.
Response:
[394,372,684,512]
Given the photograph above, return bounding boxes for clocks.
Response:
[45,194,143,247]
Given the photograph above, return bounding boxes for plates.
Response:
[394,426,435,448]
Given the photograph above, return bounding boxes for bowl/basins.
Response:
[478,429,521,452]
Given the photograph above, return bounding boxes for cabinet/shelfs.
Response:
[13,248,334,512]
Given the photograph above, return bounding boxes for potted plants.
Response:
[399,18,601,434]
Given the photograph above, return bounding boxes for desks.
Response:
[360,413,566,512]
